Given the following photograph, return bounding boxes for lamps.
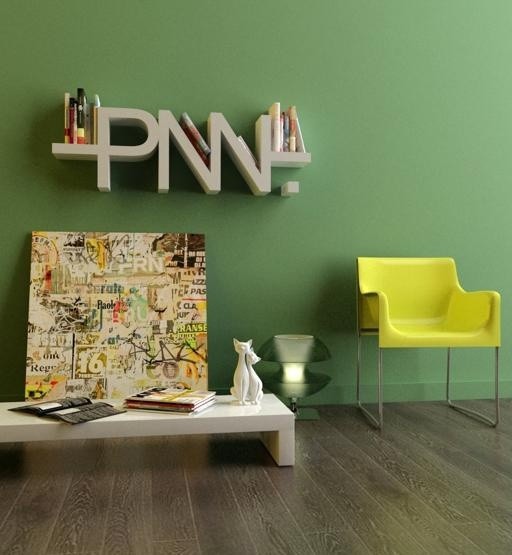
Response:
[258,333,332,421]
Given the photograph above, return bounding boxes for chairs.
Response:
[354,257,502,431]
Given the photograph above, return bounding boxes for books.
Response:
[180,111,211,156]
[8,396,128,425]
[237,135,261,169]
[63,85,101,145]
[177,119,209,173]
[266,101,306,153]
[125,386,217,415]
